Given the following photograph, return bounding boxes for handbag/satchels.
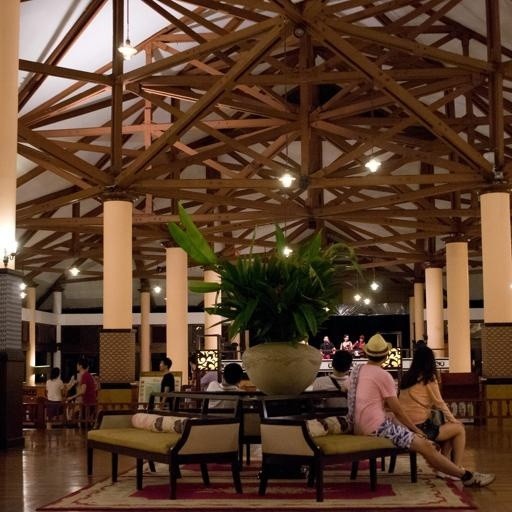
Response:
[428,407,444,429]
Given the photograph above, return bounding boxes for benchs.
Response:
[172,373,484,480]
[84,402,245,499]
[257,412,421,504]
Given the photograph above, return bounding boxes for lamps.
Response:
[152,261,164,295]
[198,347,219,371]
[361,78,383,174]
[117,0,139,65]
[352,260,380,305]
[67,242,81,278]
[1,238,19,268]
[381,347,403,369]
[276,30,300,190]
[281,193,293,259]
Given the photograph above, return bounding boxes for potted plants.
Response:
[168,197,377,399]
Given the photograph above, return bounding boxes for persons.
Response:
[396,345,465,480]
[200,363,254,414]
[304,350,351,395]
[185,356,198,403]
[318,333,368,359]
[159,357,179,410]
[45,359,96,428]
[347,334,497,489]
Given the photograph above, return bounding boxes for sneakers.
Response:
[463,471,496,489]
[435,468,462,482]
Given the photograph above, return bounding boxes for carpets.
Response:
[32,448,478,509]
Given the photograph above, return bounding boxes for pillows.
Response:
[132,410,163,433]
[158,412,198,434]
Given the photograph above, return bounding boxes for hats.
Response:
[362,332,394,358]
